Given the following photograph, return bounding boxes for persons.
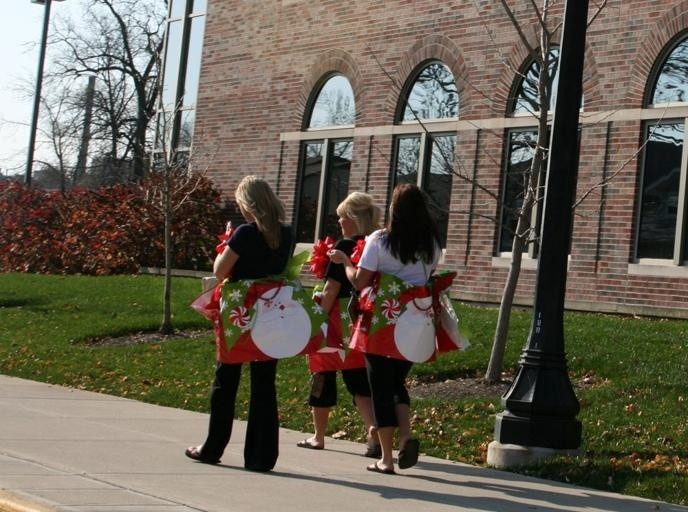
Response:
[185,175,296,472]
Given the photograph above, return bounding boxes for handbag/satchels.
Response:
[189,249,470,373]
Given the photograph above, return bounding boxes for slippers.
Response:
[185,446,221,463]
[297,438,323,449]
[365,438,419,474]
